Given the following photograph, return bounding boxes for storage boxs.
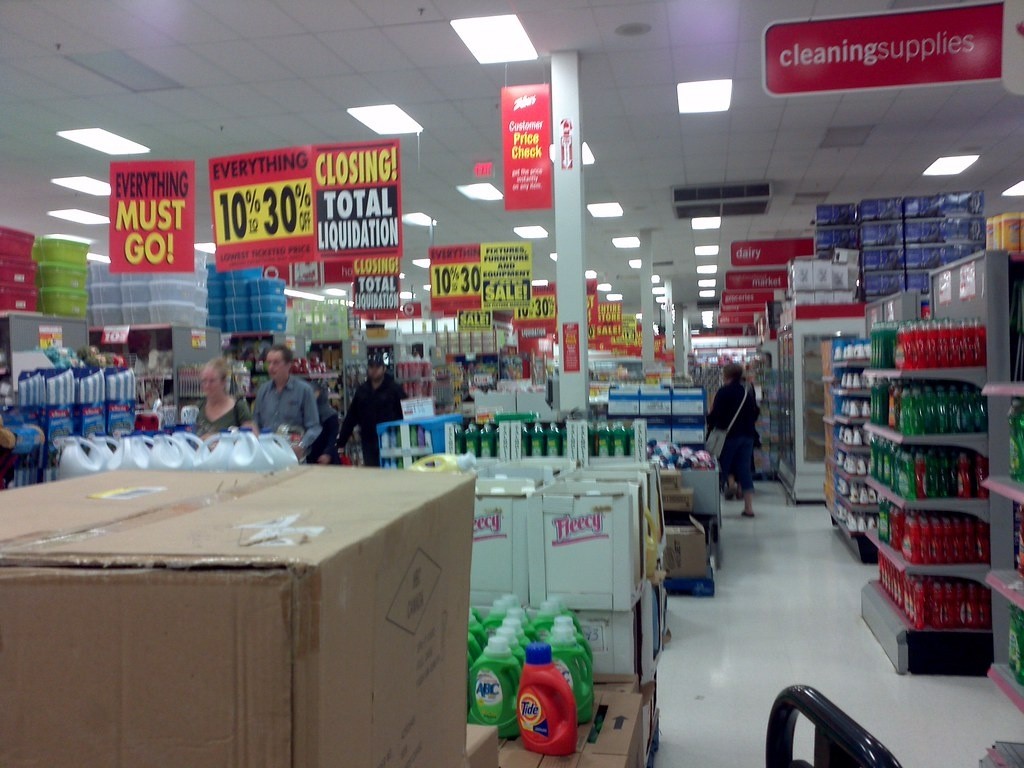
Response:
[0,224,722,768]
[786,189,987,306]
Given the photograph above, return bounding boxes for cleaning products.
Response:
[870,316,987,369]
[1017,504,1024,580]
[878,548,992,630]
[1008,600,1024,686]
[466,594,593,755]
[59,427,298,480]
[870,433,989,500]
[877,498,990,565]
[1006,399,1024,484]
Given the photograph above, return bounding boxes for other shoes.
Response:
[726,486,736,500]
[742,512,754,518]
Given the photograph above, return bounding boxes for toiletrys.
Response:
[870,377,988,435]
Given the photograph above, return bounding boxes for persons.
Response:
[336,353,409,468]
[723,373,762,501]
[251,343,322,464]
[192,356,259,454]
[706,363,760,517]
[303,378,343,466]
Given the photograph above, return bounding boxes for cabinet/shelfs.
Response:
[310,338,368,412]
[858,248,1024,679]
[366,343,437,400]
[91,323,258,412]
[980,382,1024,714]
[833,289,930,567]
[0,314,90,404]
[408,340,464,413]
[820,375,840,527]
[222,331,345,455]
[449,351,523,384]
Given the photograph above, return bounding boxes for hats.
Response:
[367,352,388,366]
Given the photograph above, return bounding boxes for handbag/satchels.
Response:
[705,427,727,460]
